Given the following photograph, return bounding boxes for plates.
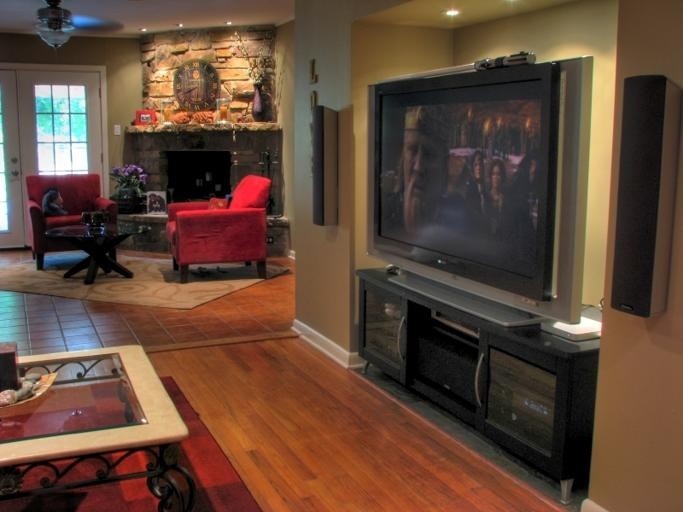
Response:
[0,371,58,409]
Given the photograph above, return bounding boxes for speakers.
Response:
[611,75,683,316]
[313,105,338,225]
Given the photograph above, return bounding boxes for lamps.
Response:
[33,1,78,48]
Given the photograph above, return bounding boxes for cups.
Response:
[216,98,231,125]
[161,100,173,124]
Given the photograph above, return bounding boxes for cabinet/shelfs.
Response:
[352,266,604,508]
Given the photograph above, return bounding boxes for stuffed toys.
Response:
[42,186,69,216]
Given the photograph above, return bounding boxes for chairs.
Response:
[24,172,121,273]
[164,174,273,286]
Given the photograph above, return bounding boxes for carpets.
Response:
[1,251,290,311]
[1,375,262,512]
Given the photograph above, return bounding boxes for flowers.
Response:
[108,164,149,197]
[233,29,276,83]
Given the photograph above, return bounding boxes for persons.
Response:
[380,100,538,277]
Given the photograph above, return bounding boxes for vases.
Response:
[251,82,265,121]
[111,197,145,213]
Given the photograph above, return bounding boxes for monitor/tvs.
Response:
[367,56,593,328]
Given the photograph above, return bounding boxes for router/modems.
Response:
[541,314,603,340]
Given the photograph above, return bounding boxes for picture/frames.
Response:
[136,109,158,127]
